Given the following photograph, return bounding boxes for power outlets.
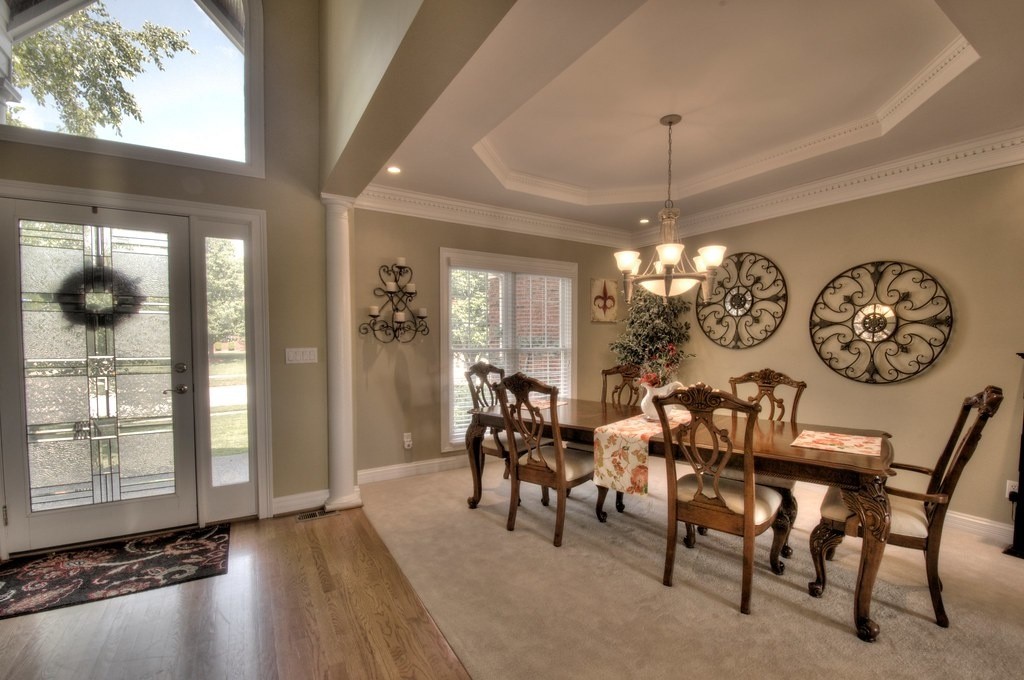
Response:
[1006,480,1018,498]
[403,432,412,442]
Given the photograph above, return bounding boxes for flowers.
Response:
[637,342,677,388]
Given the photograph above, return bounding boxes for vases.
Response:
[638,378,685,421]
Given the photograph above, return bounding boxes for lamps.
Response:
[613,114,727,308]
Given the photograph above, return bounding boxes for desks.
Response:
[464,396,895,643]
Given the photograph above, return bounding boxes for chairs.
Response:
[808,385,1004,628]
[697,367,808,560]
[492,372,610,547]
[566,363,647,513]
[650,383,791,615]
[464,362,553,506]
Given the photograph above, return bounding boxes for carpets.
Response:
[0,522,231,620]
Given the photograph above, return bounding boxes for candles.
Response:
[418,307,427,317]
[368,305,379,315]
[406,284,416,292]
[395,311,405,321]
[396,257,407,266]
[386,282,396,291]
